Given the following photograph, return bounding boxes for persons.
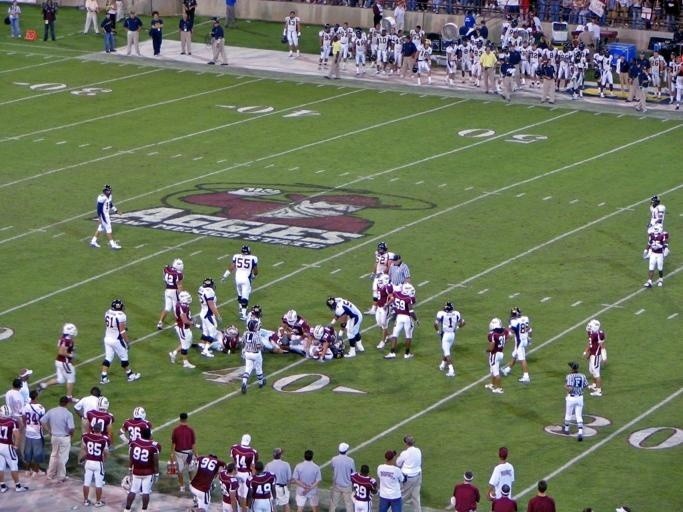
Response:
[182,0,196,29]
[435,302,465,376]
[8,0,22,38]
[158,257,266,393]
[35,322,81,404]
[364,243,419,358]
[283,11,301,57]
[225,0,240,28]
[219,245,258,320]
[488,446,519,511]
[179,13,192,55]
[527,481,556,512]
[98,298,142,385]
[451,470,480,512]
[207,17,228,65]
[649,196,666,235]
[82,0,124,52]
[583,318,607,397]
[248,298,364,363]
[644,225,668,288]
[1,368,162,512]
[90,184,122,249]
[169,411,324,512]
[558,361,589,442]
[41,0,57,41]
[307,0,682,110]
[149,11,164,57]
[483,306,532,394]
[328,434,422,512]
[123,9,143,56]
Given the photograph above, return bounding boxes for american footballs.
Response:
[489,489,495,500]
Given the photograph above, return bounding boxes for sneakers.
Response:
[562,425,569,433]
[0,469,186,512]
[88,243,122,250]
[644,281,654,286]
[578,434,582,440]
[657,280,662,287]
[36,383,48,395]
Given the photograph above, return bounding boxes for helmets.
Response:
[651,196,660,208]
[489,306,601,332]
[0,397,146,420]
[652,224,663,235]
[62,323,78,338]
[172,242,453,338]
[110,300,124,311]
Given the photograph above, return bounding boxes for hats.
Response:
[102,183,113,192]
[19,368,33,377]
[240,434,251,446]
[385,450,397,460]
[499,447,508,459]
[338,442,350,452]
